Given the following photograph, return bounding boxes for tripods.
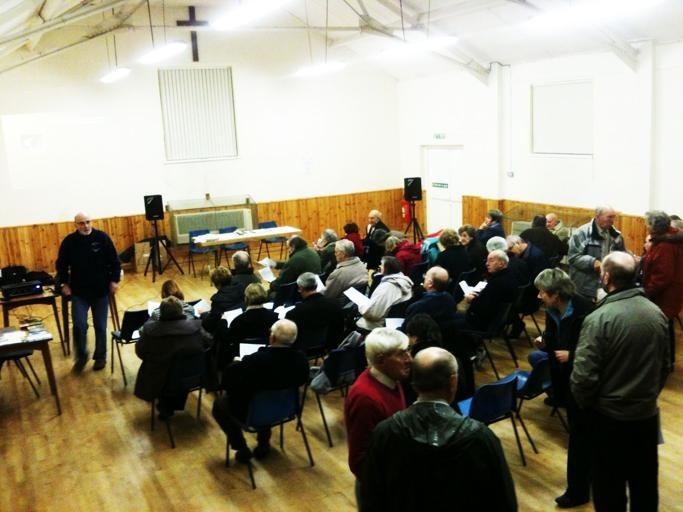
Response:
[404,205,425,245]
[143,223,185,280]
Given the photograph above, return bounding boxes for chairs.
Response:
[218,226,252,271]
[188,229,218,279]
[256,221,288,261]
[0,325,41,399]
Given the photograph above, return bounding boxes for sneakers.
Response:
[474,319,590,508]
[73,359,268,463]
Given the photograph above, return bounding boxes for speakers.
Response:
[403,177,422,202]
[143,195,164,221]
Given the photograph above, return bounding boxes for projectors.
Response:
[2,280,44,299]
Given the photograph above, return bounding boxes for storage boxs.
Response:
[134,239,168,272]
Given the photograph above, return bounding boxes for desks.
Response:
[60,285,123,356]
[0,323,62,415]
[0,286,66,356]
[191,225,303,286]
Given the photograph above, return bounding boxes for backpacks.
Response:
[420,238,440,265]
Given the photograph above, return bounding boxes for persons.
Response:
[55,213,120,371]
[131,205,683,512]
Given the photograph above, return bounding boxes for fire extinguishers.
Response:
[400,197,410,225]
